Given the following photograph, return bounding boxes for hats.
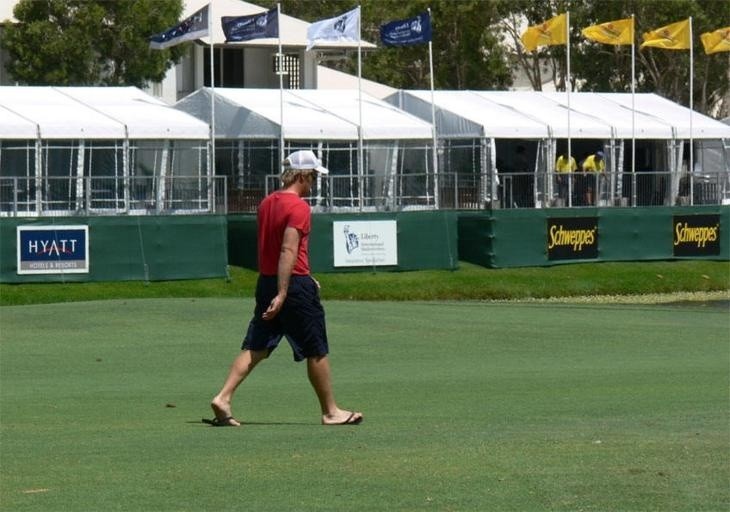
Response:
[285,150,330,174]
[596,152,605,160]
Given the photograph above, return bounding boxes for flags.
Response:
[306,7,362,51]
[380,9,434,48]
[146,2,212,51]
[639,18,693,51]
[220,4,281,45]
[520,12,569,51]
[582,17,635,46]
[699,27,730,55]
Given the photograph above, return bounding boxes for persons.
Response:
[581,150,607,206]
[200,149,363,427]
[555,149,578,201]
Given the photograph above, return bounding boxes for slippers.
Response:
[202,417,236,426]
[334,412,363,424]
